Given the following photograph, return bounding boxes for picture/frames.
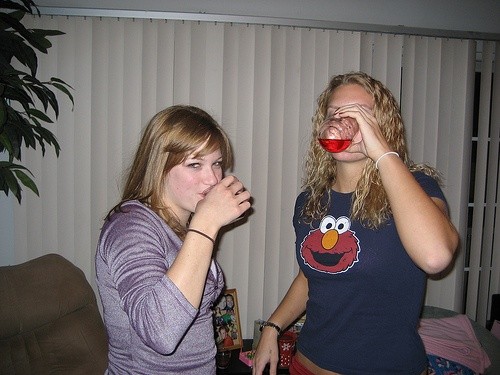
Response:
[212,288,243,351]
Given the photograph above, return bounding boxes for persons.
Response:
[95,104,251,375]
[252,71,459,375]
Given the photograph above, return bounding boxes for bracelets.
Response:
[376,152,399,169]
[186,229,215,245]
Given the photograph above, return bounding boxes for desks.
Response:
[213,339,289,375]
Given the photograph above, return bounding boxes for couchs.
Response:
[0,254,110,375]
[419,306,500,375]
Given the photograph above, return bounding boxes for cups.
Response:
[277,331,296,367]
[318,118,360,153]
[201,185,245,226]
[217,346,231,369]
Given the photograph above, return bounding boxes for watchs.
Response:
[259,321,282,336]
